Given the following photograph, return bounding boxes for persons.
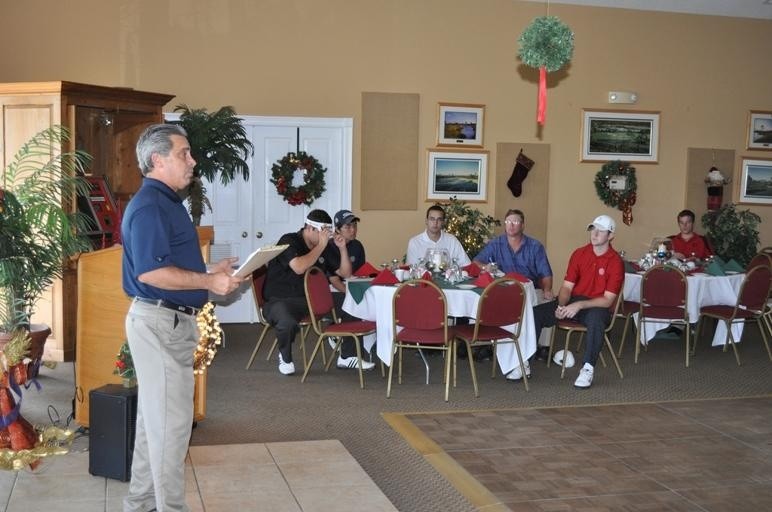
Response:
[120,120,254,512]
[326,209,367,350]
[506,214,625,389]
[476,211,556,364]
[405,204,470,272]
[264,208,376,375]
[665,207,711,264]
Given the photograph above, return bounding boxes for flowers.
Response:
[271,151,328,209]
[594,160,637,207]
[112,343,137,389]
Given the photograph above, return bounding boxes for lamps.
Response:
[609,91,637,104]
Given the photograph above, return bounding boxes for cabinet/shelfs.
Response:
[0,80,176,362]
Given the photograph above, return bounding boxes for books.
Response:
[233,244,289,278]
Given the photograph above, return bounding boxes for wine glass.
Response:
[382,256,500,284]
[617,248,722,274]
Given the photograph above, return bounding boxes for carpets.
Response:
[381,394,772,511]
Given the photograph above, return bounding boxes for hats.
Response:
[587,215,616,233]
[334,210,360,227]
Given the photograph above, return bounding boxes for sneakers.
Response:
[327,336,376,370]
[278,351,295,375]
[506,363,531,380]
[574,367,594,388]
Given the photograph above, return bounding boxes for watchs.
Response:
[481,265,486,272]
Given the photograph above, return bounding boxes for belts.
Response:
[137,297,198,316]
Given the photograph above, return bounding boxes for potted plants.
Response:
[0,124,97,379]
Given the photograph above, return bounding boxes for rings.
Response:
[324,232,327,233]
[322,231,325,232]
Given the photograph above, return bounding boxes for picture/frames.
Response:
[435,101,486,149]
[579,108,662,165]
[735,155,772,207]
[423,147,490,203]
[745,109,772,151]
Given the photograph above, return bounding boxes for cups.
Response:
[394,269,405,282]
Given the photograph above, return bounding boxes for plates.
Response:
[456,284,477,289]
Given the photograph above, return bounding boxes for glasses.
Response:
[504,220,521,225]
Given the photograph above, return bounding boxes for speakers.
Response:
[88,382,138,482]
